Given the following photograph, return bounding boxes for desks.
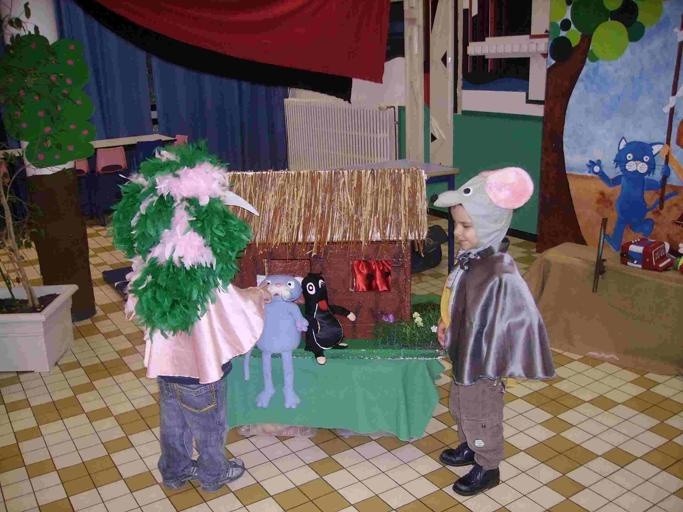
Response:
[352,160,459,281]
[0,136,180,237]
[519,242,683,377]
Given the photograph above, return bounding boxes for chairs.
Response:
[174,134,188,146]
[73,154,92,220]
[93,146,130,226]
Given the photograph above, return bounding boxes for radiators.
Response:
[284,98,396,171]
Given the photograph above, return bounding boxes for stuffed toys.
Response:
[302,273,356,364]
[242,275,309,409]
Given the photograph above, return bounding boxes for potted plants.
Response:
[0,152,79,376]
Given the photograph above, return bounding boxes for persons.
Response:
[431,167,557,496]
[107,139,272,491]
